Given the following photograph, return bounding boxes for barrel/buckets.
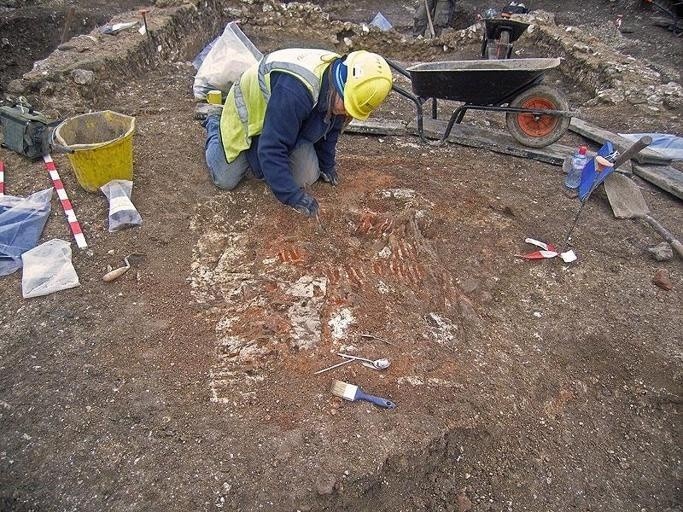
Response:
[49,109,137,194]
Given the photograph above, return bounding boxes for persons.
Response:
[201,48,394,217]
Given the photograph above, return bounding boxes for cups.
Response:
[205,91,221,105]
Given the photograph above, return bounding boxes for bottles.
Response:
[564,147,587,189]
[489,9,494,20]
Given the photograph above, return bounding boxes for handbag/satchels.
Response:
[1,96,53,161]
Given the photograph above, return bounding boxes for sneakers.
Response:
[207,107,223,117]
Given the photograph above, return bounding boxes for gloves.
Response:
[293,192,319,218]
[319,167,341,186]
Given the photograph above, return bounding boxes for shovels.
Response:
[604,172,683,267]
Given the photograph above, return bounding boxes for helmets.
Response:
[342,50,393,122]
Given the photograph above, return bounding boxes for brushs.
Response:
[331,379,396,411]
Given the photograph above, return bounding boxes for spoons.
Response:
[361,334,397,348]
[336,352,391,370]
